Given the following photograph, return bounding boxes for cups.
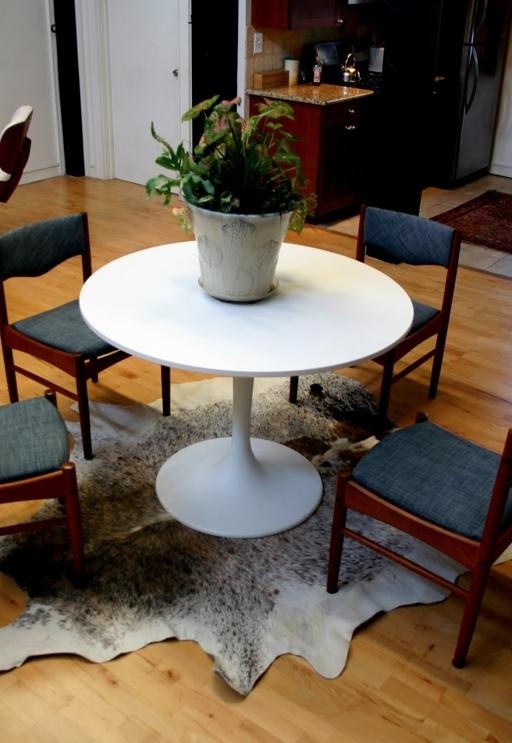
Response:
[284,58,299,86]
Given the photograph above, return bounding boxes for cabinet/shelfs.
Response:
[246,96,372,226]
[251,0,348,32]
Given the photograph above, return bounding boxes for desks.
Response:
[77,240,414,539]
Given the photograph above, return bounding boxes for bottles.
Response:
[313,65,321,86]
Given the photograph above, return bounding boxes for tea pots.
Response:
[341,53,362,83]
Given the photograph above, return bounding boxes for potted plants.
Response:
[143,94,320,305]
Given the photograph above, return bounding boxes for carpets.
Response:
[0,373,511,699]
[429,189,512,255]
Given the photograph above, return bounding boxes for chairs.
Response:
[325,409,510,670]
[289,205,460,422]
[0,389,83,577]
[1,213,171,460]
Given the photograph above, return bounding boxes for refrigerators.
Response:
[422,1,511,191]
